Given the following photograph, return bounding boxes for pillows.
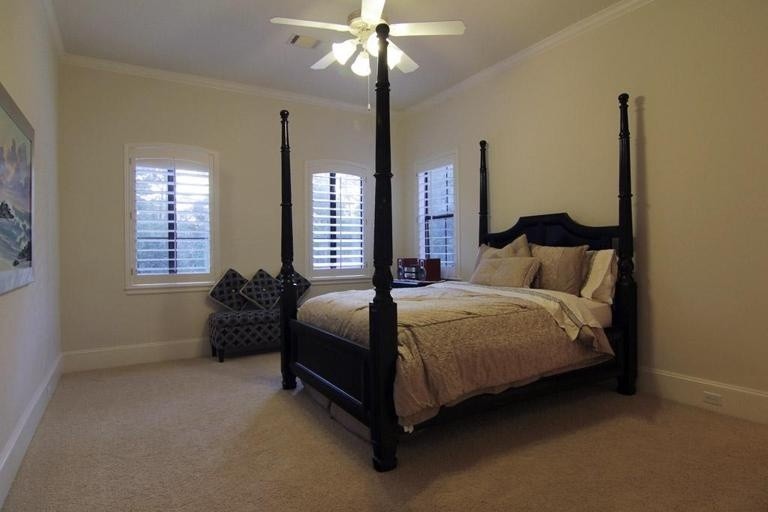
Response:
[239,269,282,311]
[208,267,250,312]
[275,269,312,306]
[470,234,620,305]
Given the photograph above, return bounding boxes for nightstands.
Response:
[392,279,444,288]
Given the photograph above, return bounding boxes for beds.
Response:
[278,22,638,473]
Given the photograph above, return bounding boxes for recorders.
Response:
[397,258,441,281]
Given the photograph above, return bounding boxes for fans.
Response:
[269,0,466,74]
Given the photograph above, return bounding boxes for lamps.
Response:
[332,32,402,78]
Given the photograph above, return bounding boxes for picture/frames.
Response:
[0,80,37,297]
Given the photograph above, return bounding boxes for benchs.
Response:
[209,305,303,362]
